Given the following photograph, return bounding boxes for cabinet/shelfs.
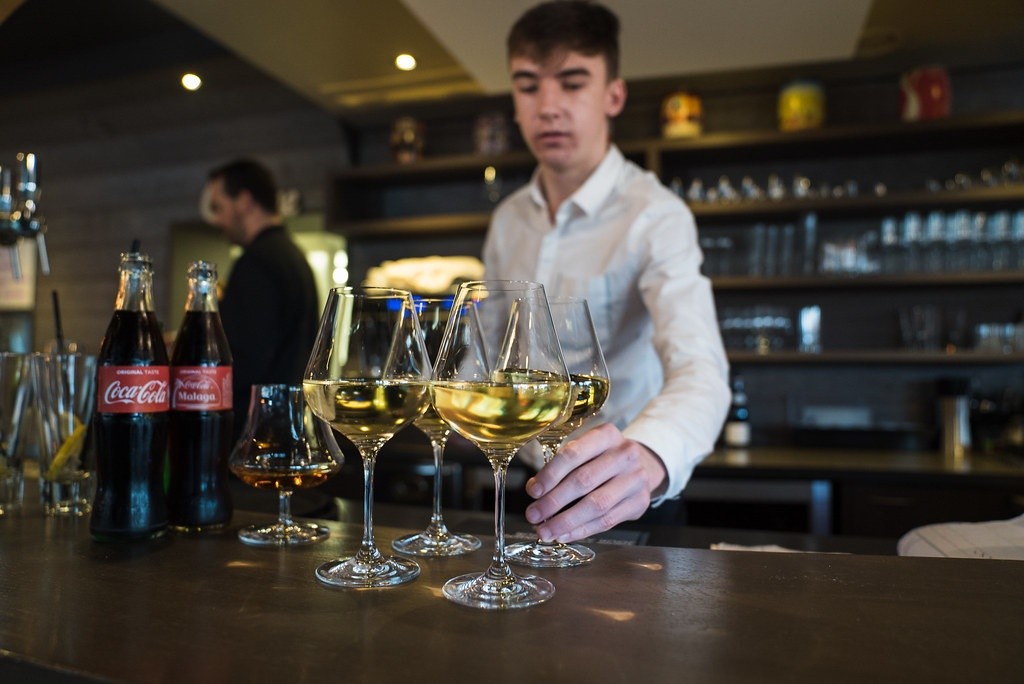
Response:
[325,38,1024,437]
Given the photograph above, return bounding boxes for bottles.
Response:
[724,381,750,451]
[89,254,168,538]
[175,264,235,529]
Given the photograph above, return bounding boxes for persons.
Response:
[444,0,731,543]
[206,156,339,521]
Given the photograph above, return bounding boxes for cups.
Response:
[29,354,97,514]
[0,353,35,513]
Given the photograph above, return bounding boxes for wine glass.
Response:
[228,385,344,549]
[498,299,608,568]
[431,283,571,610]
[305,287,430,590]
[383,301,483,556]
